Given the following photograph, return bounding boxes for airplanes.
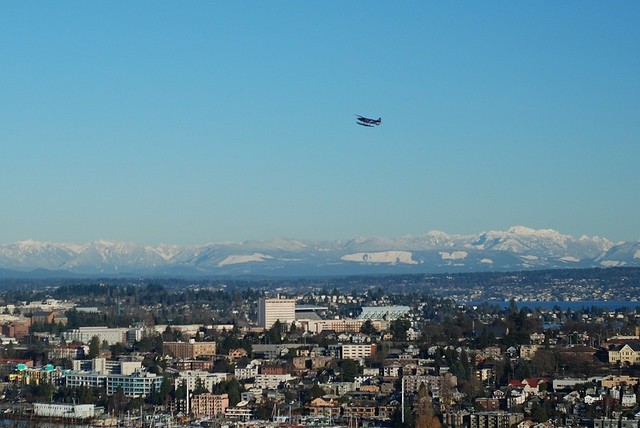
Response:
[354,114,381,127]
